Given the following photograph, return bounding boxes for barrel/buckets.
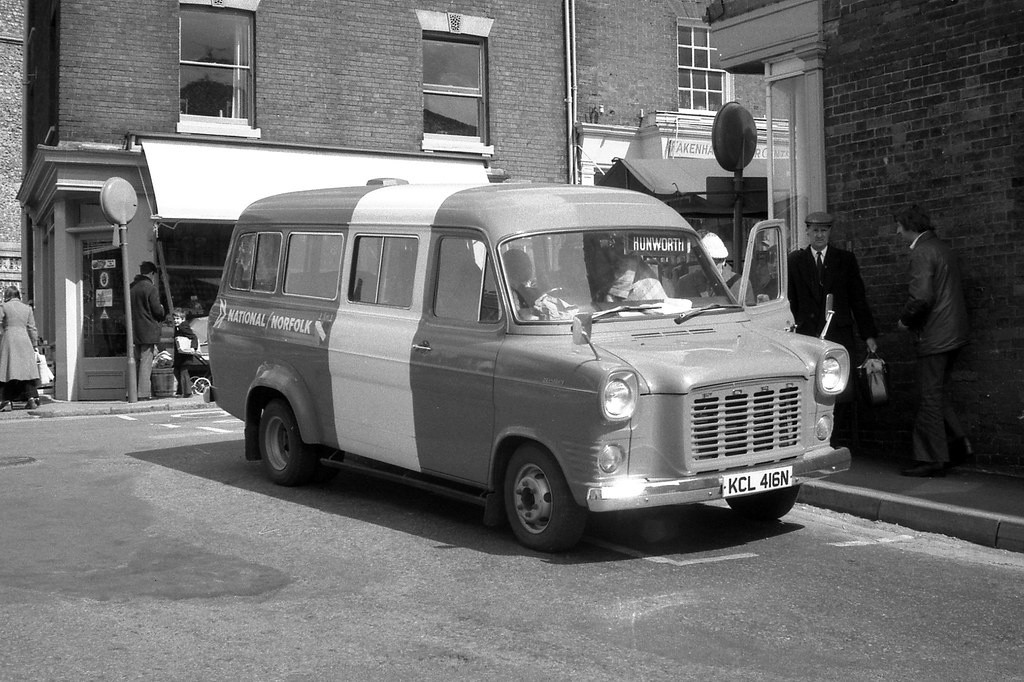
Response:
[151,368,179,397]
[856,350,888,402]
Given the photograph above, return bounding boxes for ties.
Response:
[817,252,823,281]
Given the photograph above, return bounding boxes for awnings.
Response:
[619,153,798,204]
[141,140,492,222]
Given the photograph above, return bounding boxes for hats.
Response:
[702,232,729,258]
[805,212,834,225]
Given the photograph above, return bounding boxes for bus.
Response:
[205,177,853,553]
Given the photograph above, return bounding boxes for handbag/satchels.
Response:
[35,352,54,385]
[857,351,888,400]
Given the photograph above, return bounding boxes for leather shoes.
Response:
[946,435,975,458]
[901,461,952,477]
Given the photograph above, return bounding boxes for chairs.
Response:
[503,248,533,289]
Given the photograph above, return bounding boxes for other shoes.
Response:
[137,397,159,401]
[174,393,182,398]
[126,396,128,401]
[183,393,193,398]
[25,399,36,409]
[0,401,11,412]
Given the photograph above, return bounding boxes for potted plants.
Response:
[152,358,175,397]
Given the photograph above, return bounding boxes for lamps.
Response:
[590,105,604,117]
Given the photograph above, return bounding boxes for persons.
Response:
[0,286,40,412]
[129,261,164,400]
[171,312,199,398]
[557,234,681,297]
[786,212,876,458]
[675,231,787,307]
[501,248,545,309]
[894,205,973,478]
